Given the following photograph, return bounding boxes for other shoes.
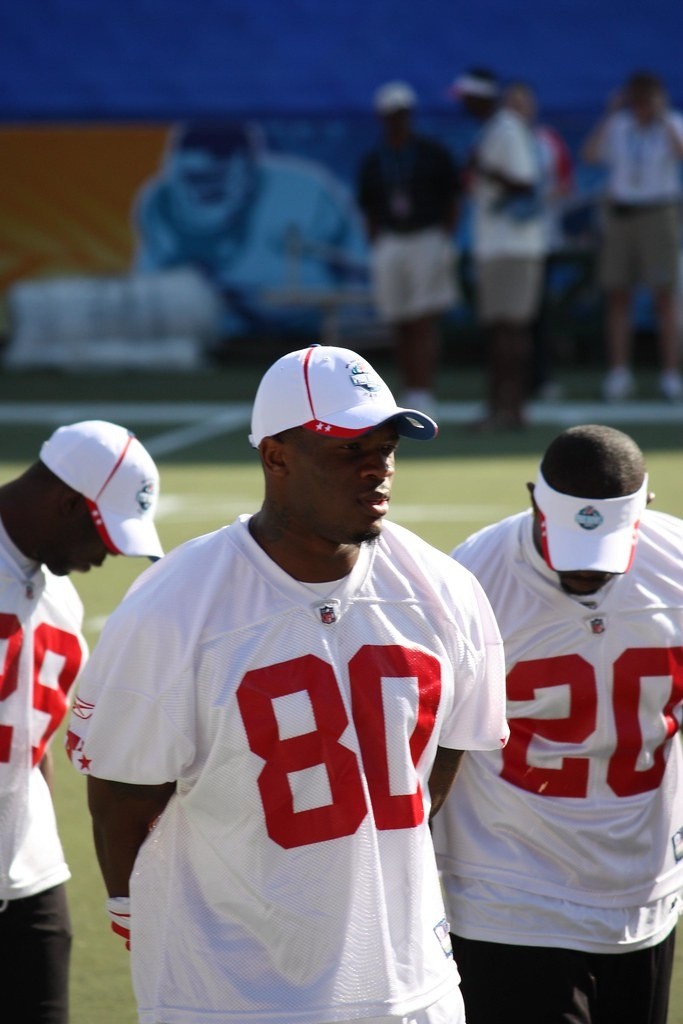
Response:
[658,373,682,398]
[606,372,637,396]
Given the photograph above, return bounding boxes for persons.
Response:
[354,67,683,439]
[67,345,511,1024]
[1,419,165,1024]
[450,426,683,1024]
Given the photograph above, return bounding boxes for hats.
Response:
[252,342,440,450]
[40,420,163,562]
[375,85,418,115]
[533,461,649,573]
[450,77,497,97]
